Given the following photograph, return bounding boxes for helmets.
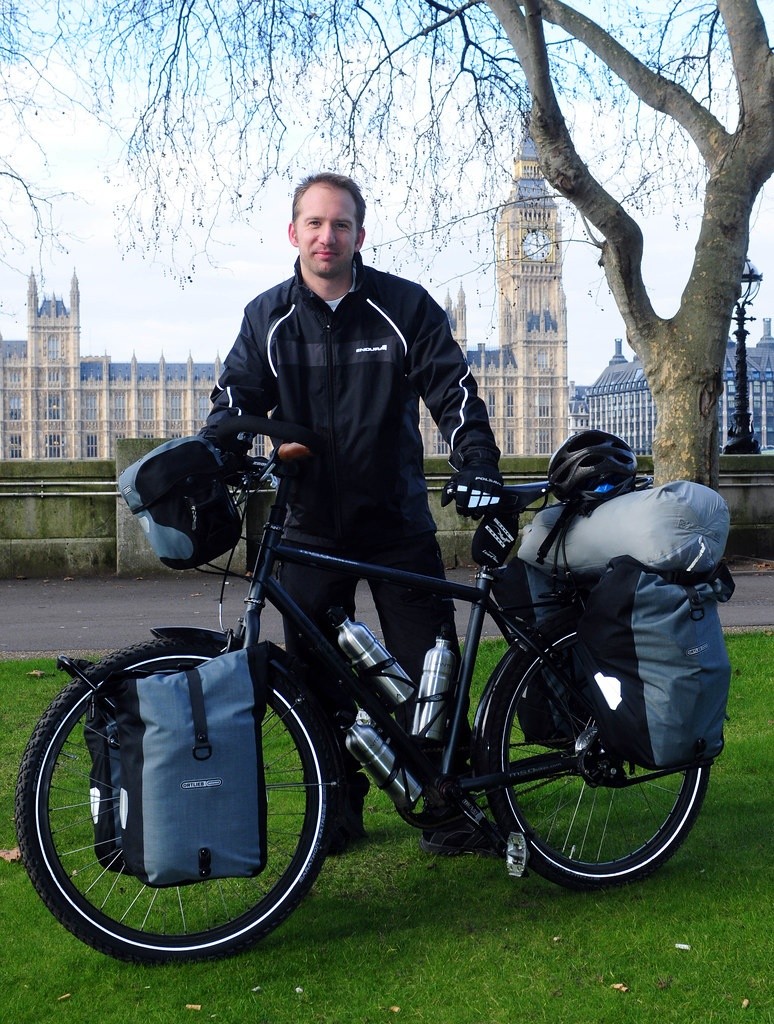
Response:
[547,429,638,512]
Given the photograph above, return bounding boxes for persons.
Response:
[204,173,507,860]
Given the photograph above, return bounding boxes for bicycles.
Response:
[16,440,721,969]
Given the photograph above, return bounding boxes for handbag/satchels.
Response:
[572,555,735,772]
[58,642,268,887]
[483,531,597,750]
[117,435,242,571]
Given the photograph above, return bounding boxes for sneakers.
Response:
[322,773,371,856]
[420,797,516,858]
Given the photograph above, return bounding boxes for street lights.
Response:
[721,256,763,452]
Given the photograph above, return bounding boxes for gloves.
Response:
[195,427,251,463]
[442,445,504,521]
